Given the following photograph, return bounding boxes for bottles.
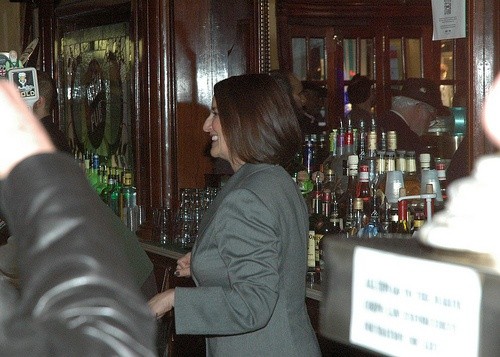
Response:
[175,187,219,249]
[71,145,136,236]
[290,117,447,284]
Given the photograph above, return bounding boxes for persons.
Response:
[377,78,443,187]
[0,69,159,357]
[147,73,321,357]
[337,74,375,131]
[205,69,308,188]
[415,74,500,256]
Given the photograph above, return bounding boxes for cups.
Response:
[152,208,171,244]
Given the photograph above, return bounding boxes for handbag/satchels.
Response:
[158,265,205,357]
